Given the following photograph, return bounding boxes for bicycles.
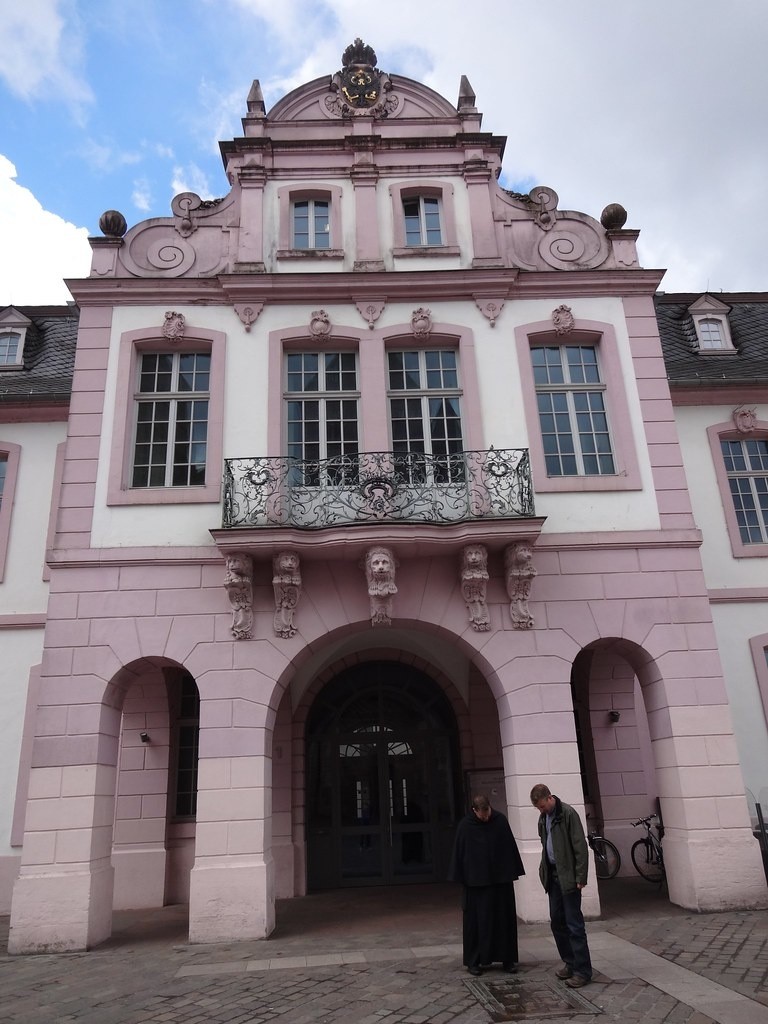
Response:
[628,811,669,885]
[585,811,621,879]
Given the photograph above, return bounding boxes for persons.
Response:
[530,785,593,987]
[447,793,525,976]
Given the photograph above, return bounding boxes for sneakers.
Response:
[556,964,591,988]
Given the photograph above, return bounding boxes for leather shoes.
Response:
[501,961,518,974]
[465,960,482,975]
